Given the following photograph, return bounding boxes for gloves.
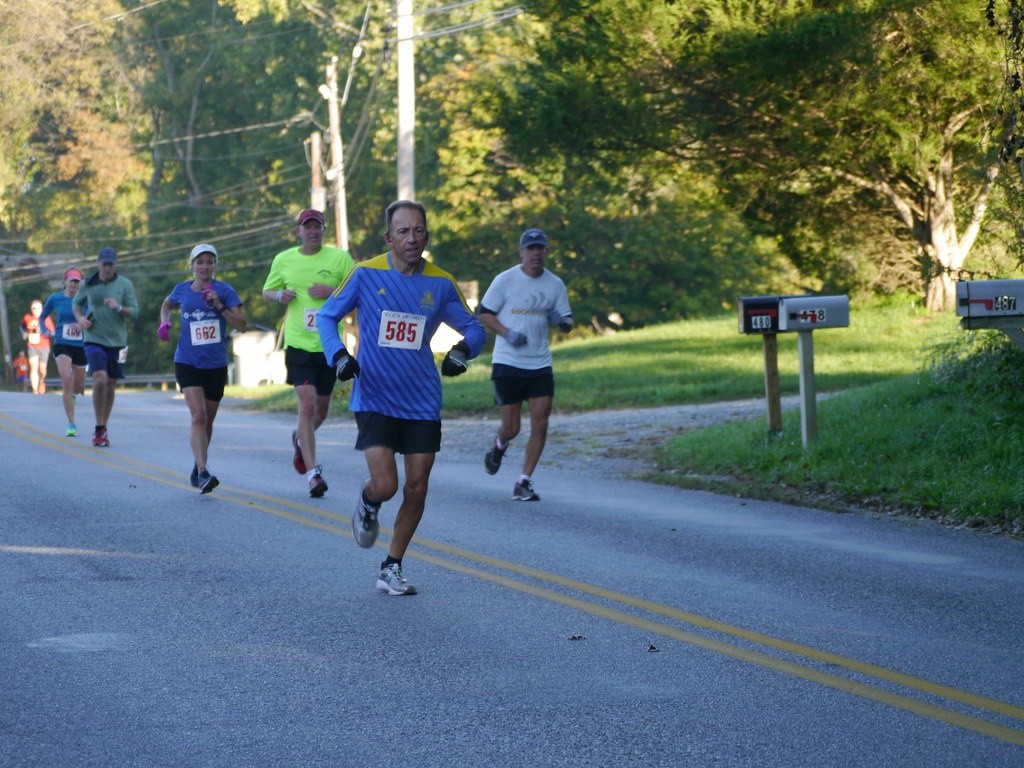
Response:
[200,285,213,305]
[545,309,564,328]
[441,341,469,377]
[504,329,528,347]
[158,321,172,342]
[333,348,360,382]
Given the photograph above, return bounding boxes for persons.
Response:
[157,244,247,495]
[313,199,487,596]
[19,299,56,394]
[39,267,89,438]
[477,228,575,501]
[262,208,357,498]
[72,248,139,446]
[13,350,30,395]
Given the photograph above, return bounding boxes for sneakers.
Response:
[376,561,417,596]
[38,382,47,394]
[307,464,329,498]
[512,479,541,500]
[290,429,306,475]
[91,426,110,447]
[64,424,77,437]
[352,477,383,548]
[483,434,509,476]
[199,467,220,495]
[71,394,77,406]
[190,460,199,488]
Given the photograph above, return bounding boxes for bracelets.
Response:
[117,304,122,314]
[218,304,227,314]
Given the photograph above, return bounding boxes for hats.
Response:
[64,268,84,281]
[98,248,117,265]
[190,243,218,263]
[520,228,549,248]
[298,210,325,224]
